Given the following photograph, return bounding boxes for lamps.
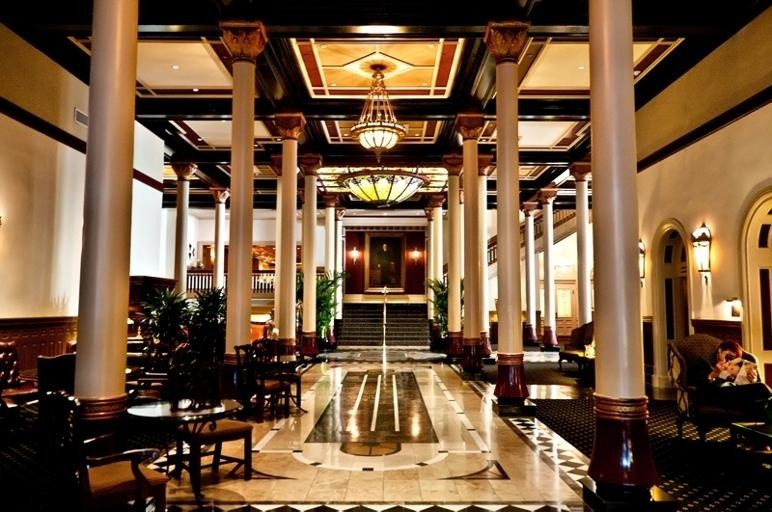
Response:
[638,239,645,289]
[692,221,712,285]
[337,170,431,208]
[348,247,360,265]
[726,295,741,316]
[410,246,422,266]
[349,64,408,165]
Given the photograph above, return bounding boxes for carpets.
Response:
[527,399,772,512]
[482,361,594,388]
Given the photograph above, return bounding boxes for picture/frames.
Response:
[196,240,302,273]
[364,232,408,293]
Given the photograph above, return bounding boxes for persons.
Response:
[695,338,772,412]
[377,243,395,286]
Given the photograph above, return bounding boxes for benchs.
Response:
[558,322,595,372]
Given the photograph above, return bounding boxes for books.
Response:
[717,353,758,385]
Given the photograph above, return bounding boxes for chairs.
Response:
[668,333,772,451]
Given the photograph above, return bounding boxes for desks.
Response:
[731,422,772,497]
